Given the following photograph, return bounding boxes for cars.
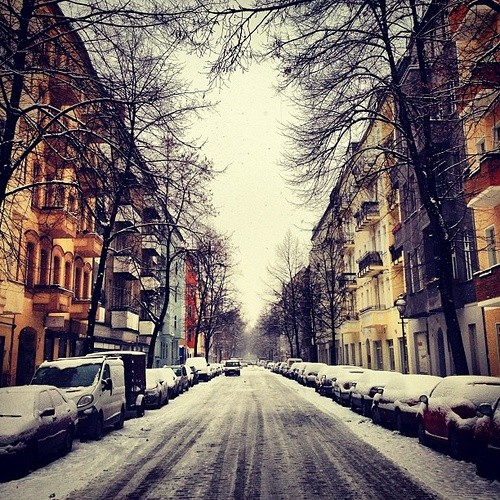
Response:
[468,395,500,480]
[0,384,80,481]
[257,356,444,438]
[415,375,500,461]
[146,357,256,411]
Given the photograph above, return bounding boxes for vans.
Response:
[86,350,147,417]
[28,353,127,441]
[223,360,242,376]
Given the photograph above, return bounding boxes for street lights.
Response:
[395,292,410,374]
